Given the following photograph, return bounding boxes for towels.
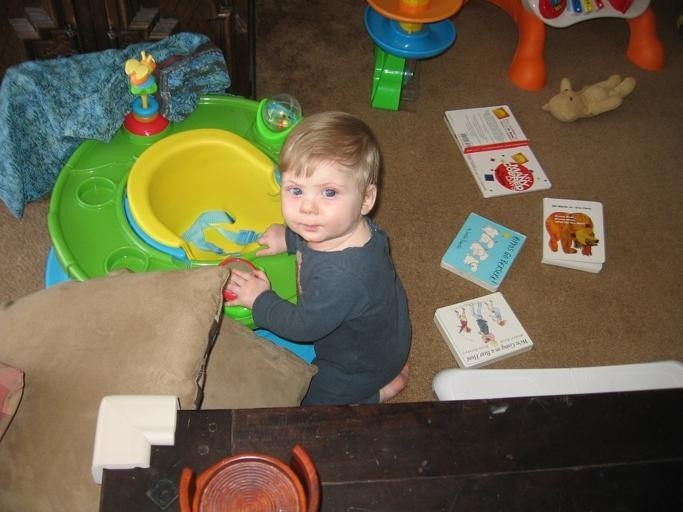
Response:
[0,30,239,222]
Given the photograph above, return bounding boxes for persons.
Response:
[219,111,415,406]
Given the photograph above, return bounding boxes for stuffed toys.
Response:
[540,73,638,124]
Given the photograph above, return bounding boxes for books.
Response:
[429,292,534,371]
[436,209,528,293]
[437,105,552,201]
[540,195,605,275]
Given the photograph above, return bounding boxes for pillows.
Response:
[0,264,230,512]
[195,312,320,409]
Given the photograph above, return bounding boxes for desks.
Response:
[87,387,683,512]
